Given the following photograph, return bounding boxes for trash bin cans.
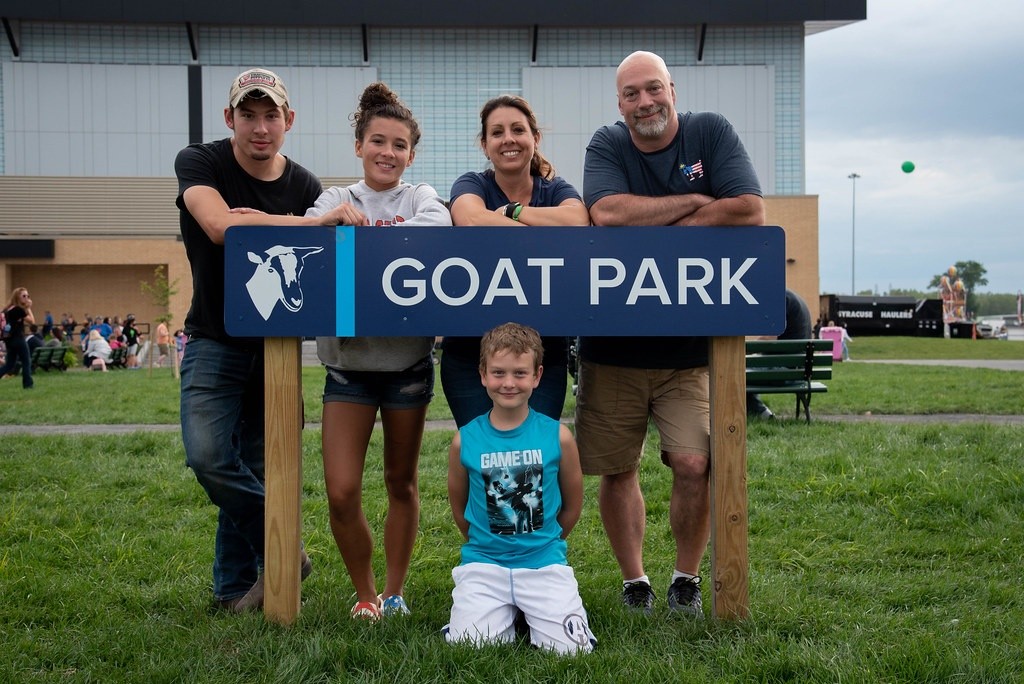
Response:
[819,327,842,359]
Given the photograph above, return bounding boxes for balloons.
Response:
[901,161,915,173]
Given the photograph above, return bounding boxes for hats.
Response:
[229,68,292,109]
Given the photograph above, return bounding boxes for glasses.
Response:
[23,295,30,298]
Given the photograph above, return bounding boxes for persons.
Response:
[441,94,590,431]
[0,287,34,389]
[24,311,78,370]
[174,68,452,623]
[814,318,853,361]
[81,313,144,371]
[441,323,598,658]
[153,319,187,368]
[747,287,812,419]
[584,50,765,617]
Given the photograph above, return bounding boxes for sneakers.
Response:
[667,575,704,615]
[234,544,313,615]
[622,581,659,613]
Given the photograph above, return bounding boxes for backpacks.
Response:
[0,306,16,339]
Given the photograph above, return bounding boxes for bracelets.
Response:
[502,201,524,221]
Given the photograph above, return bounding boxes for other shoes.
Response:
[378,593,411,618]
[351,597,384,625]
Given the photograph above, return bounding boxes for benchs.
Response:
[572,339,834,426]
[14,346,69,375]
[91,346,130,371]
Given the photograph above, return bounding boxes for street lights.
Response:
[848,172,861,297]
